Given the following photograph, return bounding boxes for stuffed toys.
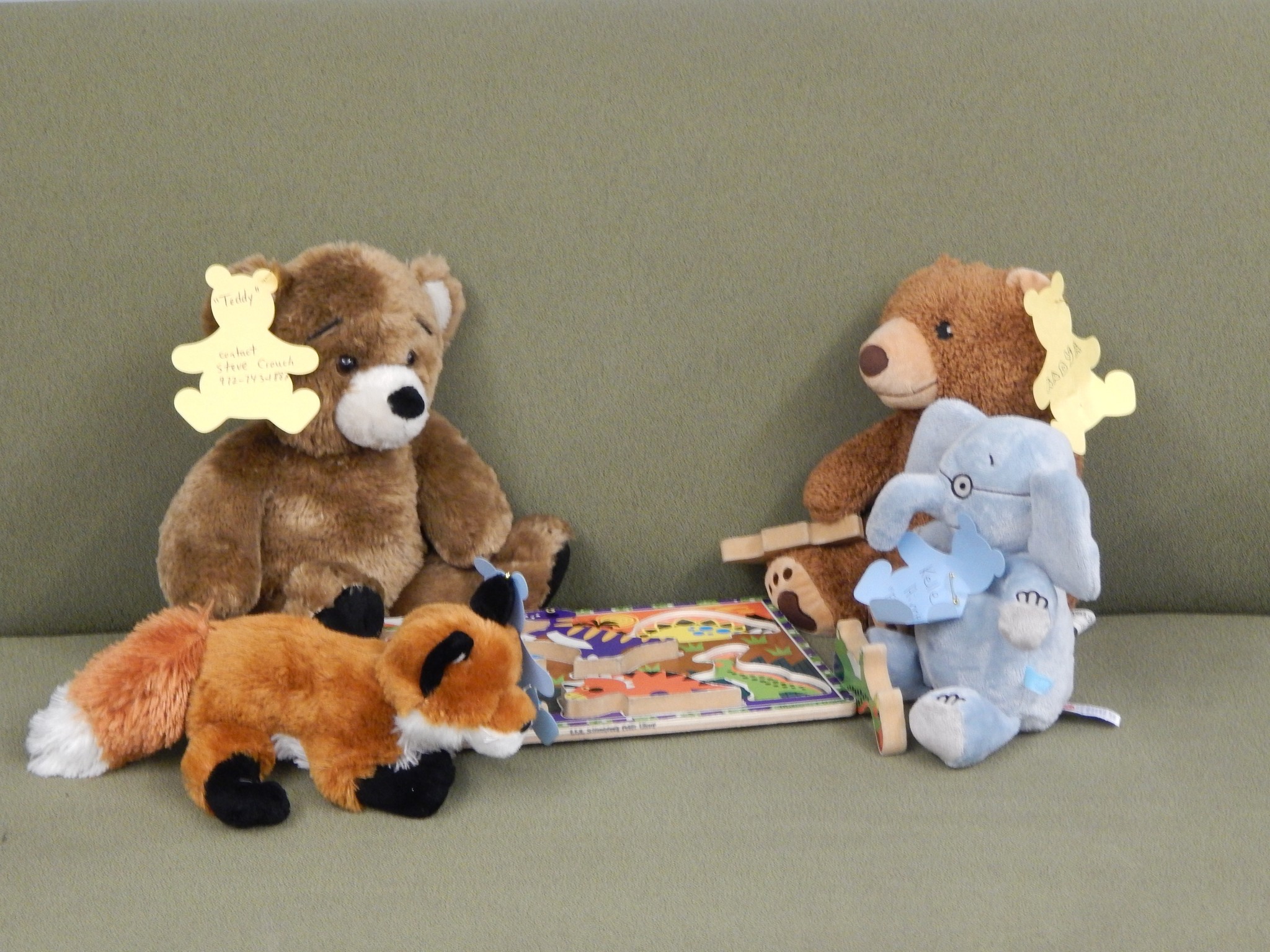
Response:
[765,253,1080,635]
[861,396,1101,768]
[156,243,572,625]
[24,573,538,828]
